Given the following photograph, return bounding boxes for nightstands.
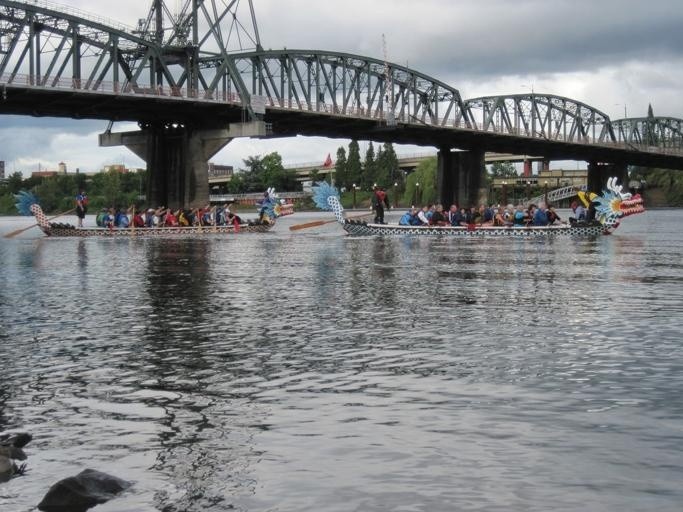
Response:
[212,207,216,233]
[230,204,240,232]
[197,210,202,233]
[109,221,113,233]
[3,208,77,237]
[289,212,376,230]
[131,204,135,236]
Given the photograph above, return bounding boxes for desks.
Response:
[311,178,645,235]
[502,181,508,206]
[416,182,420,206]
[352,183,356,208]
[545,180,548,205]
[526,180,531,200]
[394,182,398,208]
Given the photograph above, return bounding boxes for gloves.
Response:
[15,187,291,237]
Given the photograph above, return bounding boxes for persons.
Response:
[569,201,586,227]
[399,201,567,227]
[256,192,274,222]
[96,204,240,228]
[75,191,88,227]
[372,185,392,223]
